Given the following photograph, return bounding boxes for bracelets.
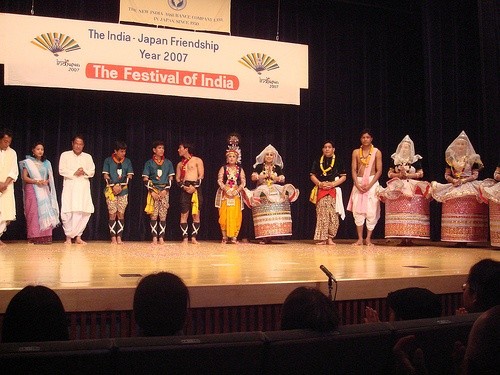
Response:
[226,189,231,193]
[318,182,321,188]
[235,190,240,196]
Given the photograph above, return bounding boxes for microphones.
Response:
[320,265,337,282]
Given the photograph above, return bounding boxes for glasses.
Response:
[462,283,473,292]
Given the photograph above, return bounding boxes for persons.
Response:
[431,131,500,248]
[309,139,346,245]
[176,142,205,245]
[279,285,340,333]
[347,129,385,246]
[0,127,19,247]
[19,142,61,245]
[378,135,434,246]
[215,136,286,245]
[142,141,175,246]
[2,285,72,343]
[102,143,134,245]
[133,272,191,337]
[364,258,500,375]
[59,135,95,245]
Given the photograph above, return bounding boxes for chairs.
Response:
[0,312,500,375]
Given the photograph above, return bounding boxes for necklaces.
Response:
[359,144,373,165]
[452,162,466,180]
[263,161,276,187]
[153,154,165,178]
[320,153,336,176]
[112,153,125,180]
[180,154,193,178]
[226,164,240,181]
